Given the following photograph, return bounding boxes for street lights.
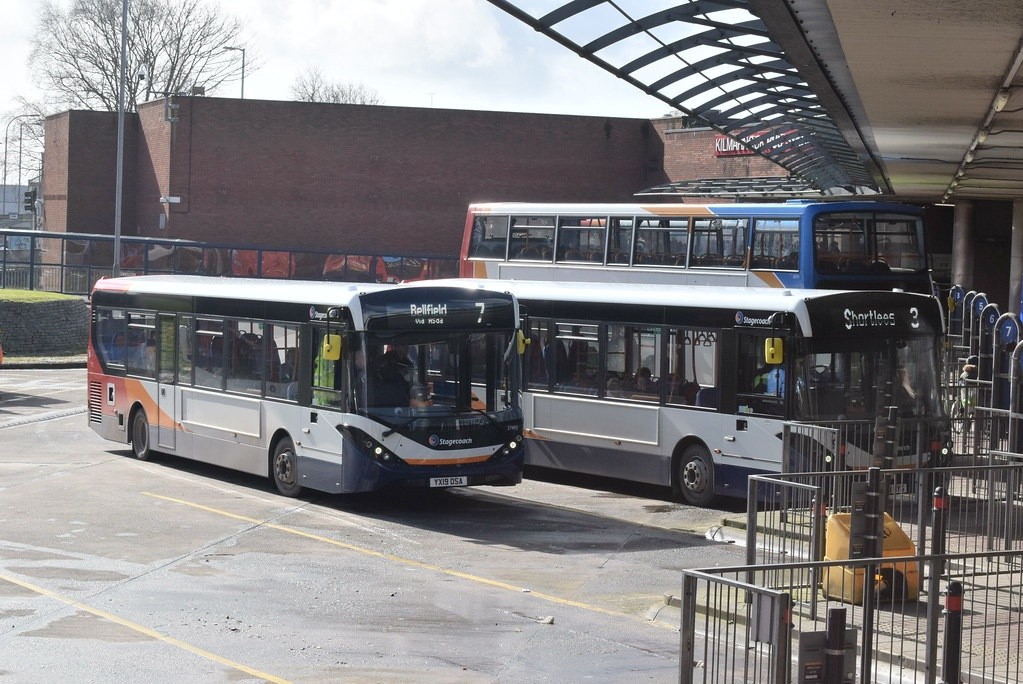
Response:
[224,46,245,99]
[0,114,42,218]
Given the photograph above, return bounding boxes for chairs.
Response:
[473,243,893,276]
[111,329,298,383]
[531,334,714,409]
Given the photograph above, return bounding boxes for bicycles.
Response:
[951,396,974,434]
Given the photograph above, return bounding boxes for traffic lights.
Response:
[23,189,35,211]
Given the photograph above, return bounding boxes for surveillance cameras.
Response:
[160,198,167,205]
[138,71,145,80]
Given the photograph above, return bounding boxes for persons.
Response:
[530,323,588,381]
[622,367,690,405]
[230,342,252,380]
[280,347,299,395]
[382,346,413,406]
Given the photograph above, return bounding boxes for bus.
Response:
[87,275,532,497]
[459,194,959,509]
